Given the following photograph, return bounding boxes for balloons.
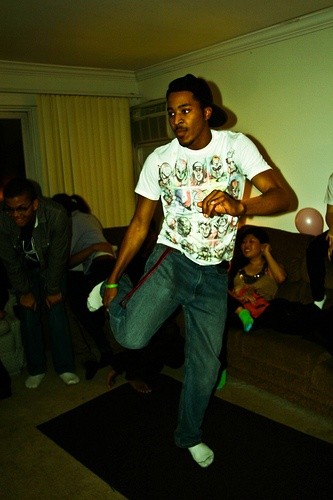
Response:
[295,207,324,236]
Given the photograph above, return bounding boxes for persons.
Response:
[306,174,333,314]
[227,225,287,333]
[87,73,288,467]
[0,178,153,395]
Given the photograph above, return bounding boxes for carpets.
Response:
[36,372,333,500]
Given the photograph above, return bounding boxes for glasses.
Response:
[4,201,33,215]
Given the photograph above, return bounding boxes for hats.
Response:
[169,73,228,128]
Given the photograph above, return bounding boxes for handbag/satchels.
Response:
[234,285,269,318]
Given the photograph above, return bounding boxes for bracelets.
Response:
[104,283,118,288]
[87,247,94,254]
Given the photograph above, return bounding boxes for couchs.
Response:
[163,225,333,443]
